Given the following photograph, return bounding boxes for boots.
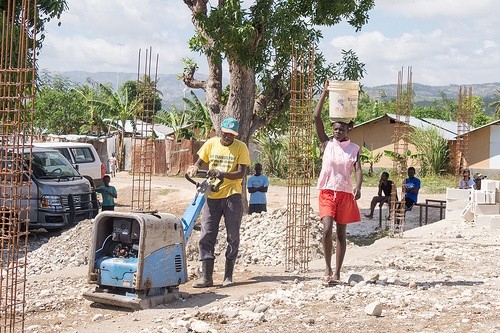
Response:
[192,259,214,288]
[223,258,235,286]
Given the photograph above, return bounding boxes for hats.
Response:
[473,172,487,180]
[220,118,240,136]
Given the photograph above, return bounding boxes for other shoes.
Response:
[364,213,373,219]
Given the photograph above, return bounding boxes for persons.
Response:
[459,169,477,189]
[470,172,487,190]
[313,79,363,284]
[247,162,270,214]
[364,172,398,221]
[92,175,118,212]
[107,152,118,178]
[395,166,421,224]
[186,117,250,288]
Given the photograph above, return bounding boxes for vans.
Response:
[0,147,97,234]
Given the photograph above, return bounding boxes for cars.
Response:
[6,142,105,190]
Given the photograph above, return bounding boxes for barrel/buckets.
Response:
[327,80,360,119]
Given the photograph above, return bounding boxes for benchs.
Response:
[380,202,446,227]
[426,199,446,224]
